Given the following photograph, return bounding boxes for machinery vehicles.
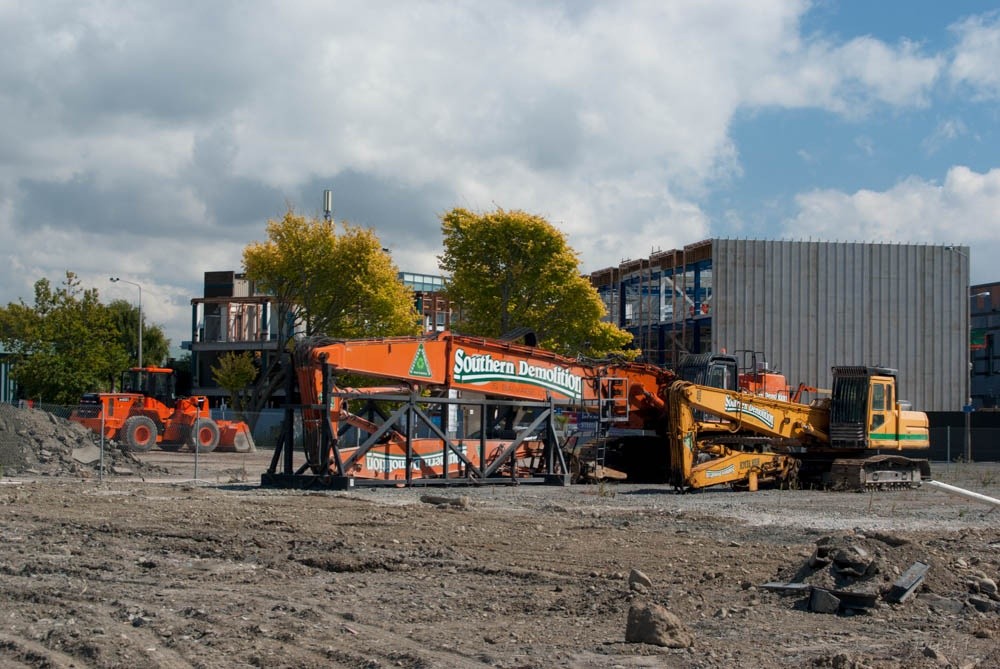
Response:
[663,366,933,494]
[259,330,832,486]
[68,363,257,455]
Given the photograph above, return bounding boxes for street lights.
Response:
[110,277,143,393]
